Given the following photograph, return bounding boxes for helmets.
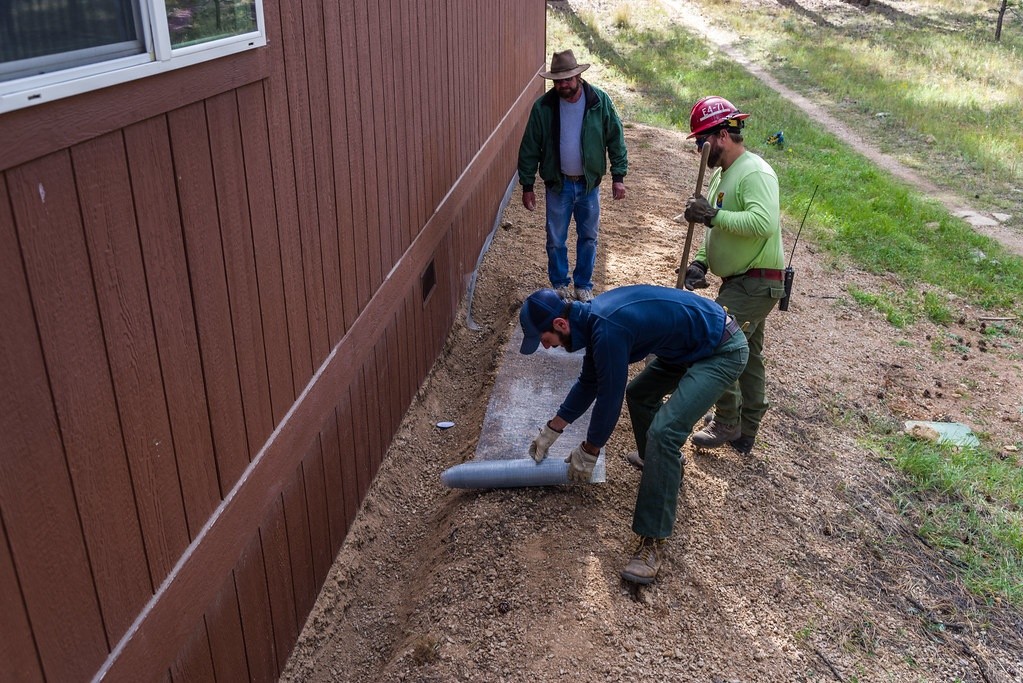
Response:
[685,96,750,140]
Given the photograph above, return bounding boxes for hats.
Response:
[519,288,566,355]
[539,49,590,79]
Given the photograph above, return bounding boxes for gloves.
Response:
[675,261,710,291]
[528,420,564,462]
[564,441,600,485]
[684,192,719,228]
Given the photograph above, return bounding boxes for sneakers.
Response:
[627,449,687,482]
[556,285,573,300]
[689,419,742,449]
[621,534,665,583]
[706,415,755,454]
[574,285,594,302]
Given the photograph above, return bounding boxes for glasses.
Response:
[695,132,717,148]
[553,76,577,82]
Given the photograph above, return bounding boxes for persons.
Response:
[674,96,784,453]
[517,50,628,302]
[519,284,750,582]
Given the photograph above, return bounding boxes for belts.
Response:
[746,268,785,281]
[566,177,584,182]
